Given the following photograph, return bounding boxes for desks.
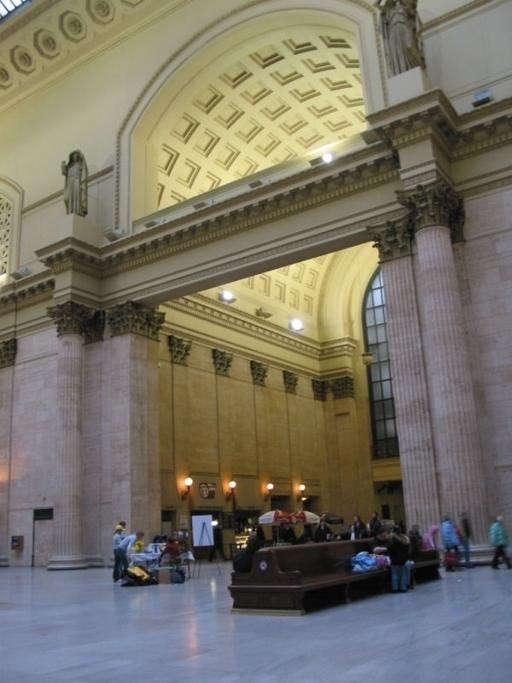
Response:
[129,550,195,579]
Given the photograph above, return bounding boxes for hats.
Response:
[116,525,126,530]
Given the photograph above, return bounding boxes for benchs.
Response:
[227,535,441,616]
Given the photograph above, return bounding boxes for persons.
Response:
[61,148,89,216]
[233,511,473,593]
[111,521,189,582]
[207,512,228,561]
[489,515,512,569]
[373,0,427,77]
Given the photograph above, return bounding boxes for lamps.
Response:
[288,319,305,332]
[266,483,274,496]
[219,290,237,304]
[299,485,306,497]
[181,477,193,499]
[227,481,237,496]
